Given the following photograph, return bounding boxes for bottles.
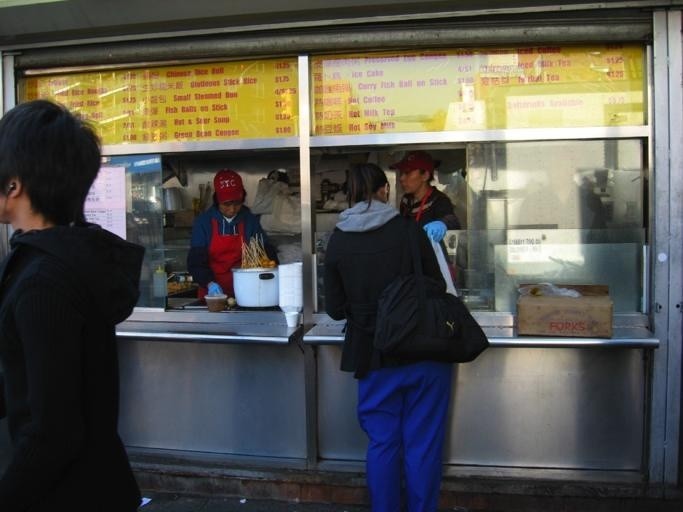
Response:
[153,265,168,297]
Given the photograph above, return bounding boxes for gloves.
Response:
[423,221,448,242]
[207,282,223,297]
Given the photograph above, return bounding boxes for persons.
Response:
[389,150,461,244]
[322,160,490,512]
[0,98,143,512]
[187,167,280,303]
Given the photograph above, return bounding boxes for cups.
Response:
[284,312,299,327]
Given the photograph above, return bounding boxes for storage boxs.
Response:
[516,283,614,339]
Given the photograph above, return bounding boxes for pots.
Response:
[230,267,279,308]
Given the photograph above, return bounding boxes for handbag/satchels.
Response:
[418,293,490,364]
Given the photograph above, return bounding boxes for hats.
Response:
[387,150,433,173]
[213,171,245,204]
